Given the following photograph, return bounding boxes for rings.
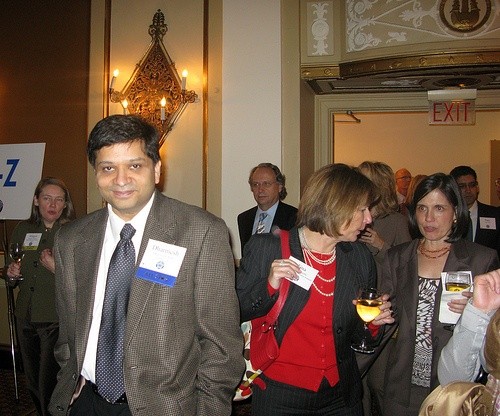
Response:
[389,310,393,314]
[370,234,372,237]
[280,260,284,266]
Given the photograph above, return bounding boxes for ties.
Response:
[94,224,137,405]
[255,213,268,235]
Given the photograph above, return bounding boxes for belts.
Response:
[83,379,128,405]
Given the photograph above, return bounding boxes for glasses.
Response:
[397,176,412,180]
[458,180,478,191]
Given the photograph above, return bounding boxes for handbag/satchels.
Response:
[231,313,280,403]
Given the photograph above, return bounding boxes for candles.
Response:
[110,69,119,87]
[123,99,128,116]
[161,96,166,121]
[181,70,188,90]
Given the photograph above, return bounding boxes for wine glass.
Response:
[8,242,26,280]
[351,287,386,354]
[443,271,472,332]
[361,222,373,246]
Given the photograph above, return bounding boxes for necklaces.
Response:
[298,229,336,296]
[420,242,451,259]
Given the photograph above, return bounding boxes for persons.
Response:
[393,169,428,216]
[437,268,500,416]
[354,171,500,416]
[47,114,246,416]
[237,163,298,258]
[4,176,77,416]
[449,166,500,255]
[356,161,413,292]
[234,163,395,416]
